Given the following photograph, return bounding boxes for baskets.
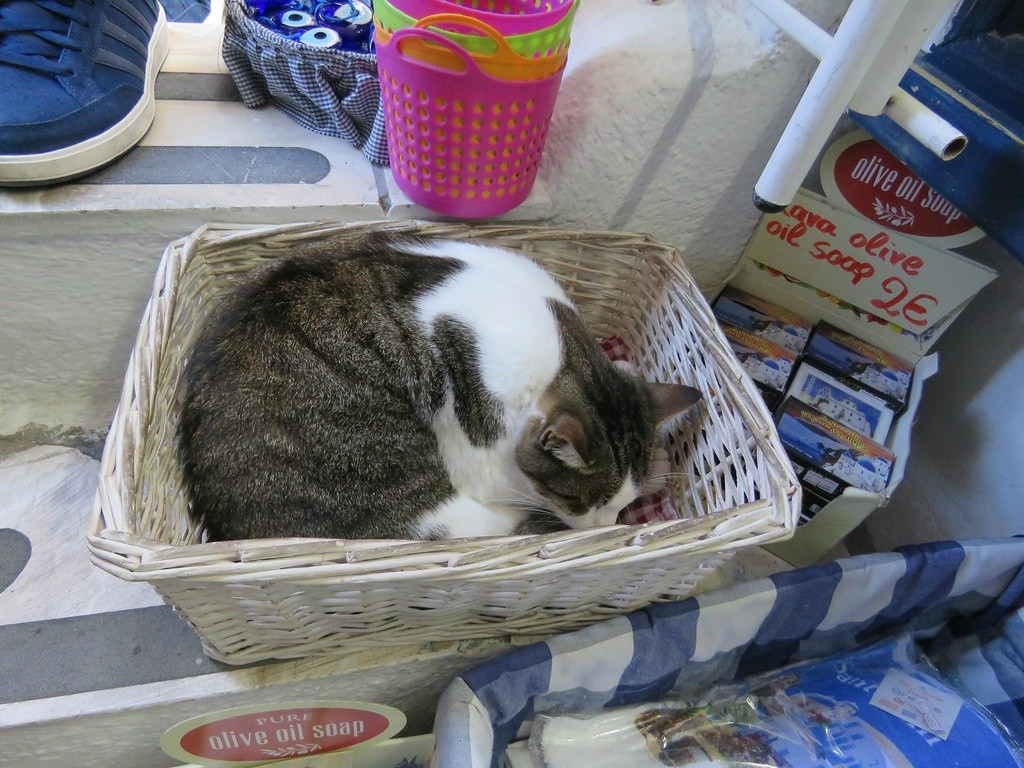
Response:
[84,217,803,666]
[372,0,581,217]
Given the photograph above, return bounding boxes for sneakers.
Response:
[0,0,171,187]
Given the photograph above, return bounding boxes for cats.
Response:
[176,229,704,545]
